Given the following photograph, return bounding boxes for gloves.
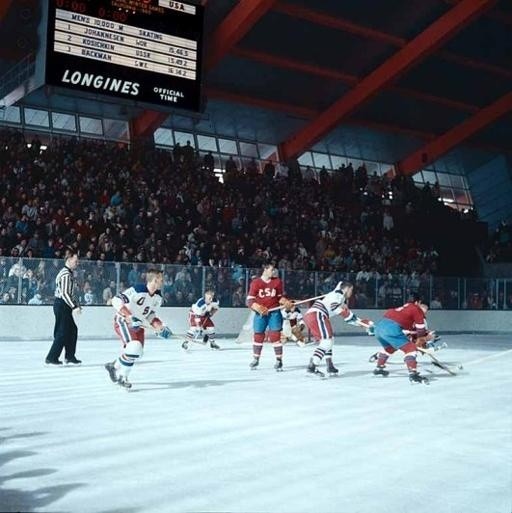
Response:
[205,312,213,317]
[250,302,269,316]
[198,327,203,335]
[278,296,294,310]
[298,323,306,332]
[359,319,375,329]
[157,327,173,341]
[131,317,144,329]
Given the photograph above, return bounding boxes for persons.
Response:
[224,146,245,308]
[303,280,372,378]
[247,261,290,372]
[43,249,81,367]
[375,167,430,298]
[106,268,172,388]
[247,155,288,260]
[290,160,375,279]
[432,181,512,312]
[0,249,68,304]
[374,299,431,385]
[282,300,306,348]
[106,136,174,267]
[81,132,104,307]
[368,316,447,359]
[183,289,222,350]
[176,137,222,288]
[0,123,79,248]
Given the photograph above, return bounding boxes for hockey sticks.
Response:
[417,346,457,376]
[185,315,208,355]
[268,281,343,312]
[438,360,463,369]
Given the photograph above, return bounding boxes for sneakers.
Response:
[294,338,306,348]
[407,369,430,383]
[248,354,260,367]
[273,357,283,370]
[103,360,118,383]
[371,364,390,376]
[209,342,220,348]
[117,376,133,389]
[368,351,381,363]
[181,340,193,351]
[326,365,340,374]
[305,358,326,378]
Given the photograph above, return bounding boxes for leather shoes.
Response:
[44,356,63,365]
[64,358,82,366]
